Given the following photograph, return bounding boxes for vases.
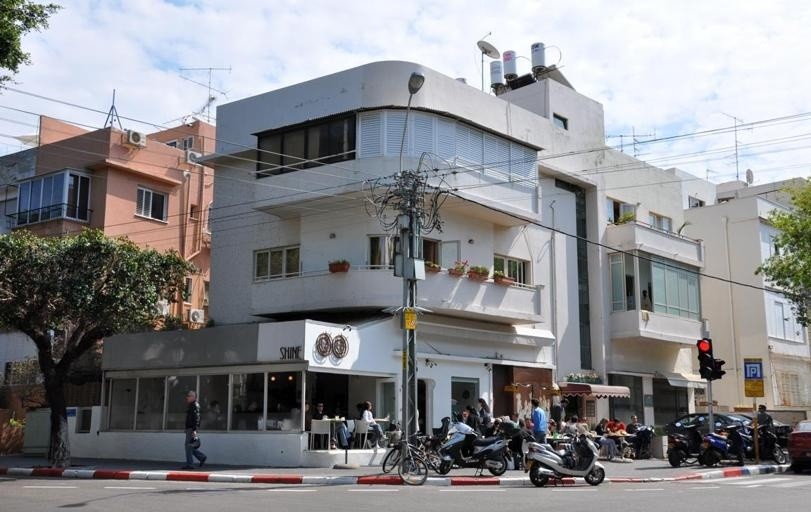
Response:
[448,269,463,276]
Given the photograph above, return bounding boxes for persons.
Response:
[757,405,773,428]
[200,399,390,450]
[181,390,207,470]
[466,398,642,471]
[641,288,651,309]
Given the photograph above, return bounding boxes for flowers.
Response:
[454,259,468,272]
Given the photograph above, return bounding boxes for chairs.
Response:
[306,419,324,450]
[351,420,376,449]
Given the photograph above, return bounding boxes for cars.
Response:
[728,410,792,450]
[663,410,754,456]
[786,419,810,474]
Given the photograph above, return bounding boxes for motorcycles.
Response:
[701,420,751,468]
[738,421,788,465]
[666,415,707,468]
[526,428,606,488]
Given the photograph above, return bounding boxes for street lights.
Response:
[394,68,429,476]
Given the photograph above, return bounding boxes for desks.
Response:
[609,435,637,459]
[546,436,602,456]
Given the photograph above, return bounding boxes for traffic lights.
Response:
[696,337,716,381]
[713,358,726,380]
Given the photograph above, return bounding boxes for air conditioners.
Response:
[150,301,169,316]
[185,150,202,167]
[126,130,147,148]
[188,309,205,323]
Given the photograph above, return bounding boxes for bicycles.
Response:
[381,408,511,486]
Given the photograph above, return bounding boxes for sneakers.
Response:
[181,456,207,470]
[374,435,389,449]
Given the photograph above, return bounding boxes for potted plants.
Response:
[424,260,441,272]
[327,258,350,272]
[467,264,489,281]
[492,270,516,285]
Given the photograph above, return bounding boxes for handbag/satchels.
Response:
[188,435,201,449]
